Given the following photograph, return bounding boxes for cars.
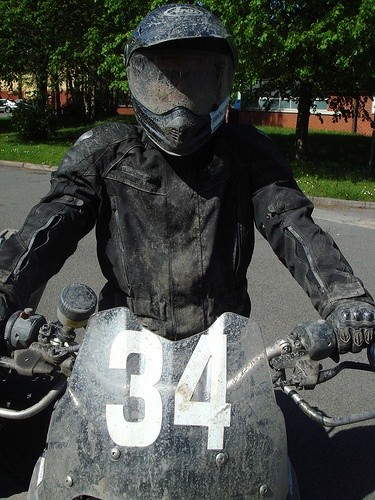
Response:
[0,98,18,118]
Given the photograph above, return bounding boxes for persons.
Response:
[0,3,375,500]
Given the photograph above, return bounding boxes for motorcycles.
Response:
[0,283,375,499]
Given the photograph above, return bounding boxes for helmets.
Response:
[125,3,238,158]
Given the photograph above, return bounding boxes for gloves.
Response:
[326,300,375,364]
